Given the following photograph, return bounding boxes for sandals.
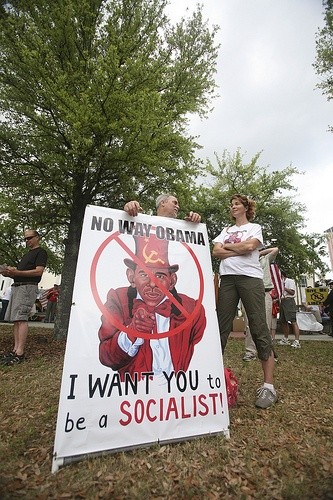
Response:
[1,351,26,365]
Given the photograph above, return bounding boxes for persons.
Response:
[276,271,301,348]
[0,230,47,365]
[212,194,278,409]
[241,248,279,361]
[124,194,201,223]
[44,284,59,322]
[319,282,333,336]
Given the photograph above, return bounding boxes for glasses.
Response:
[24,235,37,242]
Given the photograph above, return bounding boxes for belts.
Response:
[11,282,38,287]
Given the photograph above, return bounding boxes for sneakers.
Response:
[277,335,291,346]
[291,340,301,348]
[254,386,277,408]
[242,350,258,361]
